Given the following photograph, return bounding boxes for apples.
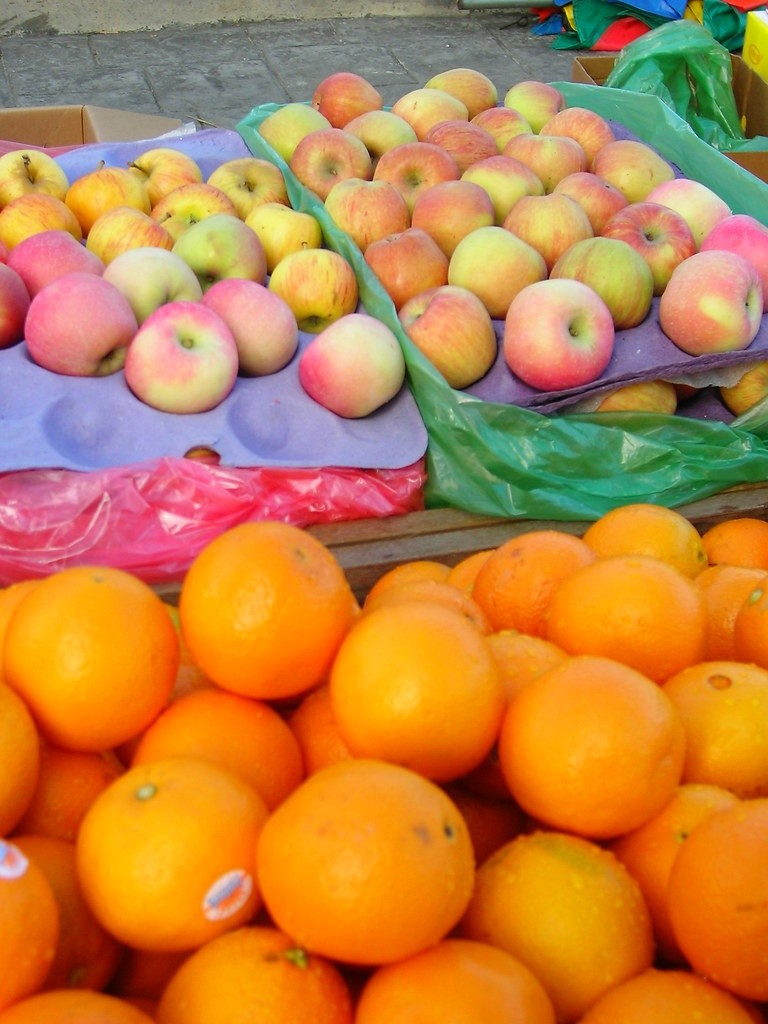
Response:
[0,67,768,458]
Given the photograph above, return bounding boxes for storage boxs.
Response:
[0,104,185,146]
[742,10,768,83]
[571,51,768,185]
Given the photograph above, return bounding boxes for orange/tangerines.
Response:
[0,503,767,1024]
